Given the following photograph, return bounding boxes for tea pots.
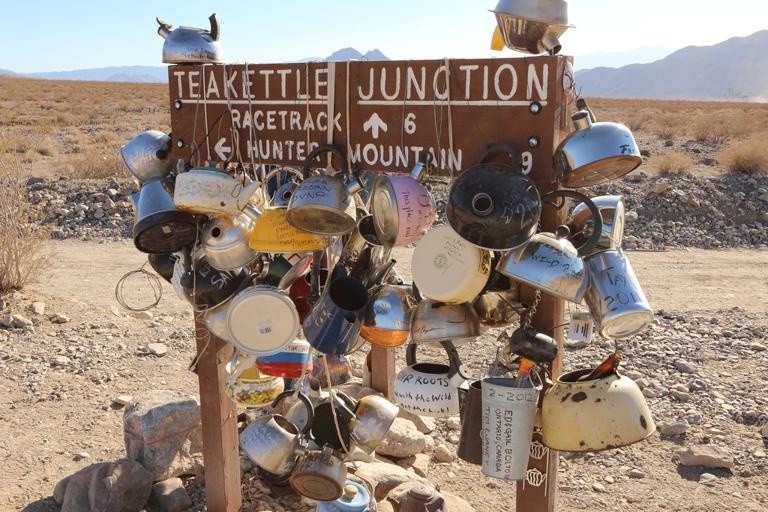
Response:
[121,109,656,512]
[155,13,224,63]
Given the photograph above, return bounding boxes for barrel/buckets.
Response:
[481,378,544,481]
[583,248,654,343]
